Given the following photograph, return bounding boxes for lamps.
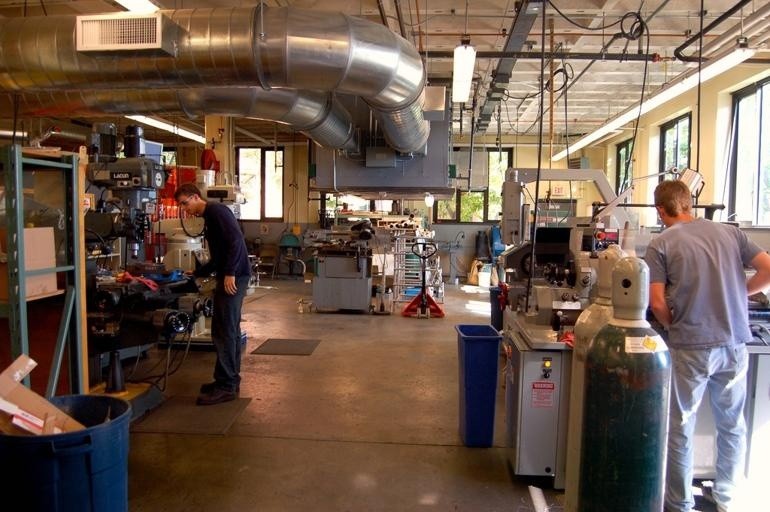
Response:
[452,4,476,104]
[547,5,758,168]
[122,116,206,149]
[103,0,166,11]
[424,192,435,207]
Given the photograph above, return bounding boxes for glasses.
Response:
[182,195,193,205]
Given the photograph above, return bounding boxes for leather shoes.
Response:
[201,381,239,393]
[196,387,237,405]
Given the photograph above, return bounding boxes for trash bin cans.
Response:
[454,324,505,447]
[489,287,503,331]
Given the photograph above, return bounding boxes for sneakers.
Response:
[701,480,726,512]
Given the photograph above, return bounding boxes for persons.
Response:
[342,203,347,211]
[174,183,251,405]
[645,180,770,512]
